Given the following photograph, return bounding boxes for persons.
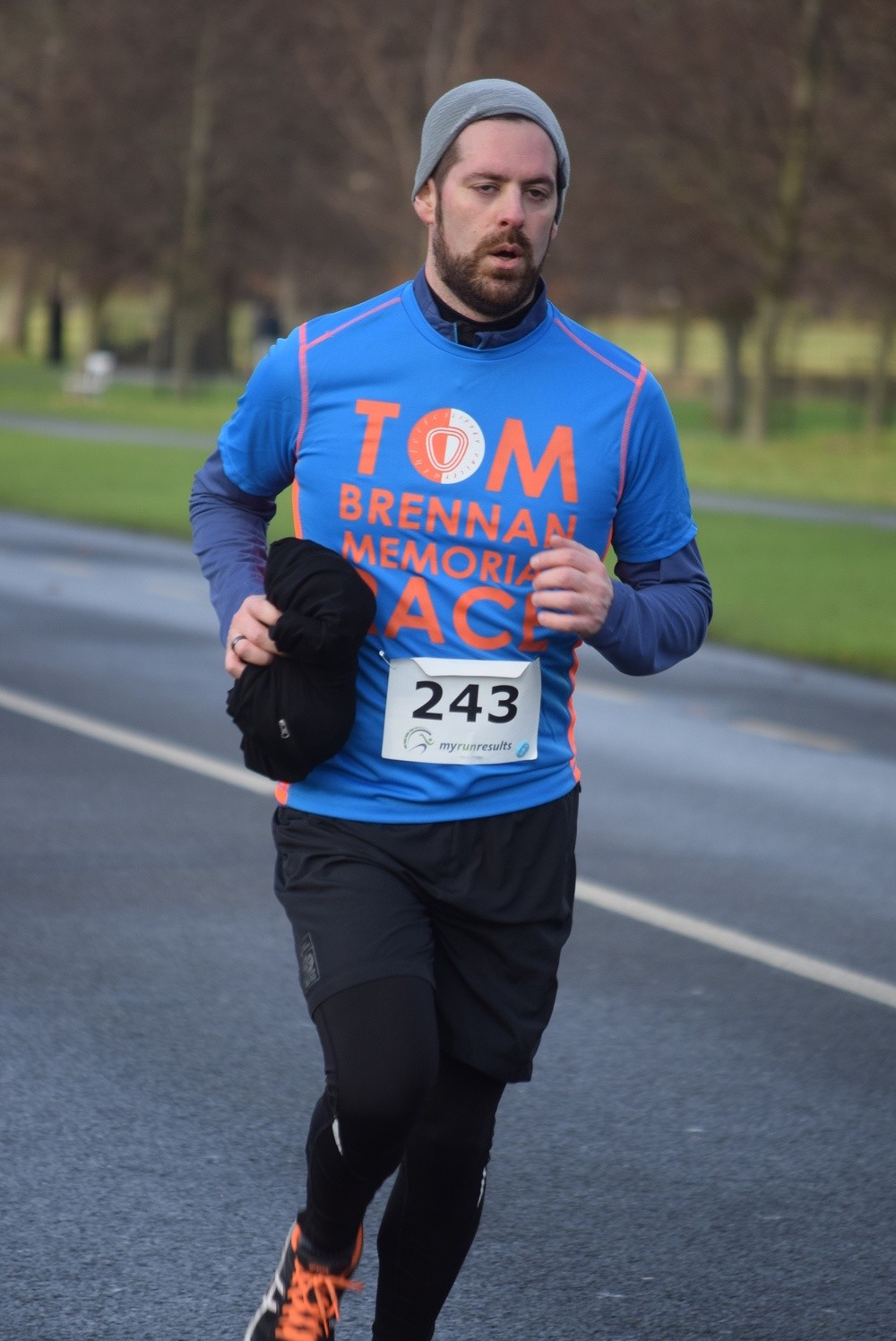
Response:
[184,78,714,1340]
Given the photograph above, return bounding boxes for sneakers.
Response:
[245,1215,363,1340]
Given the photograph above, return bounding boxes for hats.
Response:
[410,78,571,226]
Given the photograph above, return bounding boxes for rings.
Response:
[230,635,246,654]
